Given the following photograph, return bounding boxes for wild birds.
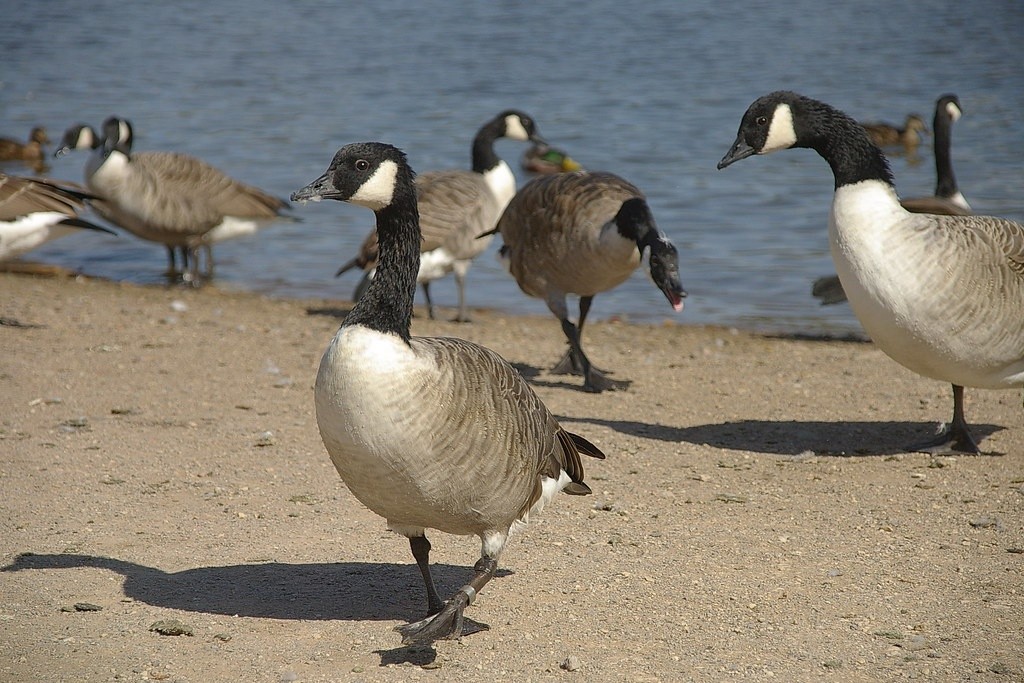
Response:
[0,116,308,297]
[334,109,587,322]
[717,91,1024,467]
[812,93,973,309]
[475,170,688,394]
[290,142,606,653]
